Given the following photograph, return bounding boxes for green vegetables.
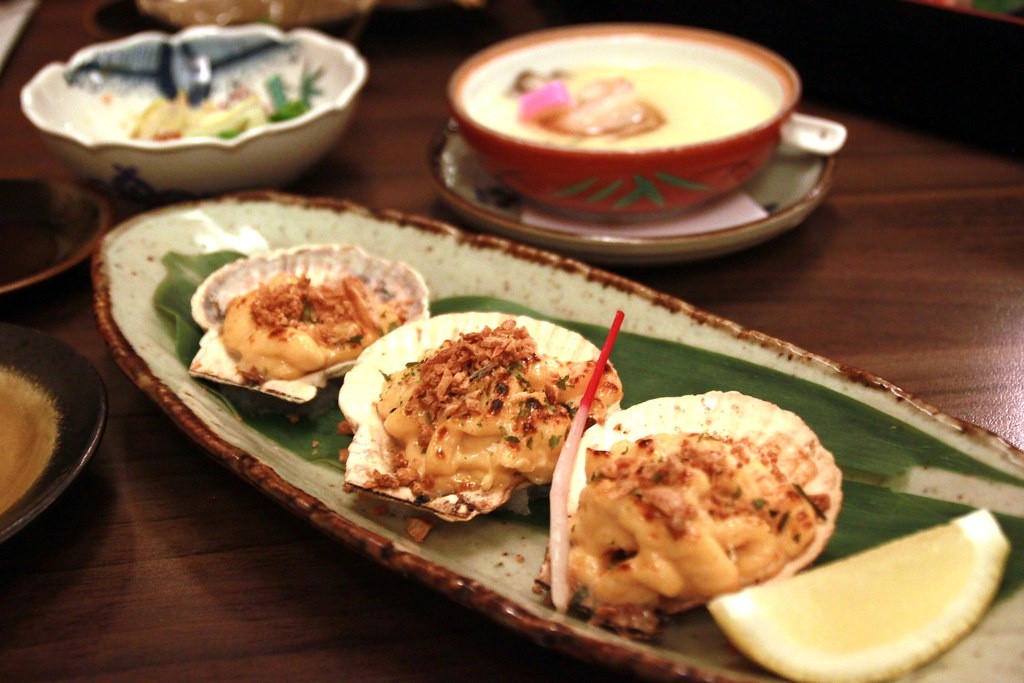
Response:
[216,58,328,139]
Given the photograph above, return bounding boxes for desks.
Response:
[0,0,1024,683]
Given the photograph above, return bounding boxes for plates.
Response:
[1,324,108,541]
[425,117,838,263]
[92,193,1023,681]
[2,172,109,303]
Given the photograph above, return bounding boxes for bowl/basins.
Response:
[19,23,368,194]
[447,23,800,213]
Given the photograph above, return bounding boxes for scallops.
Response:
[186,244,843,634]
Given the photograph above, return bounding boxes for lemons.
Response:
[706,507,1012,682]
